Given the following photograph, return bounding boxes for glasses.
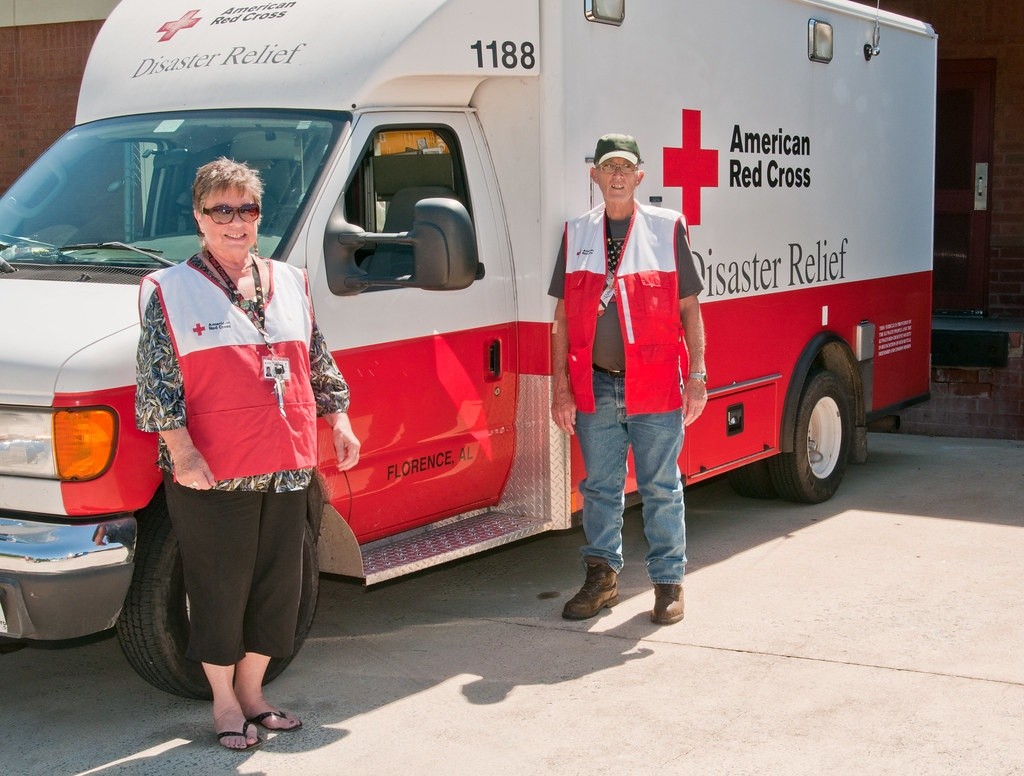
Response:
[203,204,259,224]
[595,161,638,174]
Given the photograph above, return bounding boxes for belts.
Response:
[592,363,625,379]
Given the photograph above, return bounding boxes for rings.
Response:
[191,481,198,487]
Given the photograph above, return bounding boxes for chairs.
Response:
[359,186,463,276]
[248,157,295,236]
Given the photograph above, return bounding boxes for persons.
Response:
[135,156,361,750]
[549,134,708,624]
[266,367,272,376]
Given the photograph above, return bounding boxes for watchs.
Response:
[689,373,707,384]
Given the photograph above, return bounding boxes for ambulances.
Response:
[1,0,942,706]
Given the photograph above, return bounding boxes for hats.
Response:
[593,133,640,167]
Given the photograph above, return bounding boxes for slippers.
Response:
[246,710,303,733]
[216,721,263,752]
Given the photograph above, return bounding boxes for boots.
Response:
[650,584,684,624]
[562,556,619,620]
